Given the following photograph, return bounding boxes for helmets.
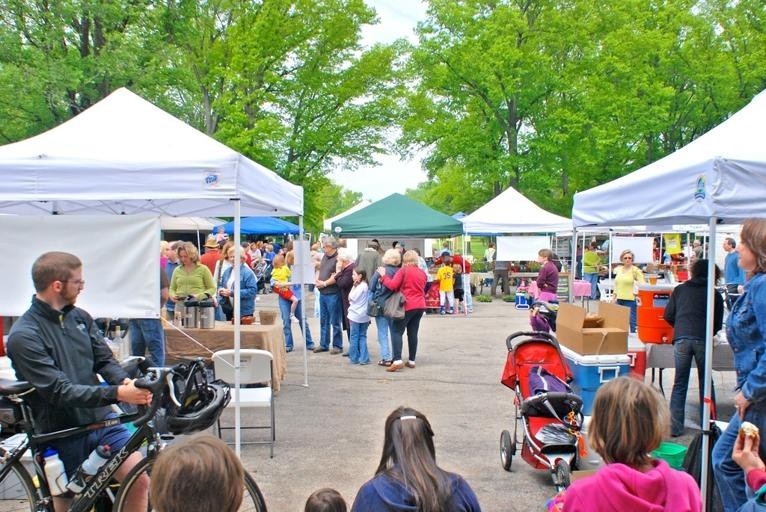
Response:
[166,379,231,435]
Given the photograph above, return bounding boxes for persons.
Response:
[433,243,473,315]
[560,376,702,512]
[7,253,149,512]
[304,406,482,512]
[483,243,559,341]
[576,236,744,335]
[162,226,427,371]
[128,267,171,366]
[711,219,766,512]
[150,433,243,512]
[663,260,724,437]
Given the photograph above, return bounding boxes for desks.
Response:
[164,320,290,396]
[631,329,736,399]
[472,271,540,295]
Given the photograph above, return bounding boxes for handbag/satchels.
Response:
[366,299,380,317]
[383,292,405,320]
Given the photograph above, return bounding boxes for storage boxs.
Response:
[626,334,646,379]
[555,301,631,355]
[559,344,630,390]
[575,389,597,416]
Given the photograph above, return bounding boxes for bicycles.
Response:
[0,355,268,511]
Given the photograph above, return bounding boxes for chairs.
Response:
[597,280,613,302]
[211,349,276,458]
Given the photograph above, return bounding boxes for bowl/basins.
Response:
[258,310,277,325]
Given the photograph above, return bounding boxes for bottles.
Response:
[663,267,675,285]
[506,261,542,275]
[67,444,113,495]
[161,299,216,330]
[40,444,71,497]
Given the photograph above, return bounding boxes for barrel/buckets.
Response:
[634,283,676,344]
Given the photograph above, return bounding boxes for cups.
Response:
[246,315,255,322]
[649,275,658,285]
[240,316,252,325]
[231,317,241,324]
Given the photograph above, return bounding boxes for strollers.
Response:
[251,257,275,295]
[530,299,560,332]
[499,330,584,495]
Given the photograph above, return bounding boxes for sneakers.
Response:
[377,359,414,372]
[308,347,348,356]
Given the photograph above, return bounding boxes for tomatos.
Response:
[232,316,255,324]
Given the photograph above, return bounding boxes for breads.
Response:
[739,421,759,441]
